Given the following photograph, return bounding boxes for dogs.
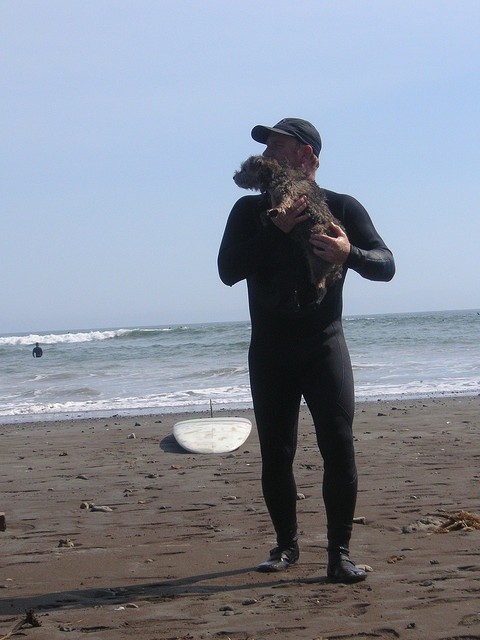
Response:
[233,155,347,312]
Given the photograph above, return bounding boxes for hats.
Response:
[250,116,322,157]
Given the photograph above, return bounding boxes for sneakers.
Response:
[327,550,368,583]
[257,540,299,572]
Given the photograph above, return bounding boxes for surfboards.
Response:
[173,417,252,454]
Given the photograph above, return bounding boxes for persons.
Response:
[32,342,43,358]
[218,119,396,584]
